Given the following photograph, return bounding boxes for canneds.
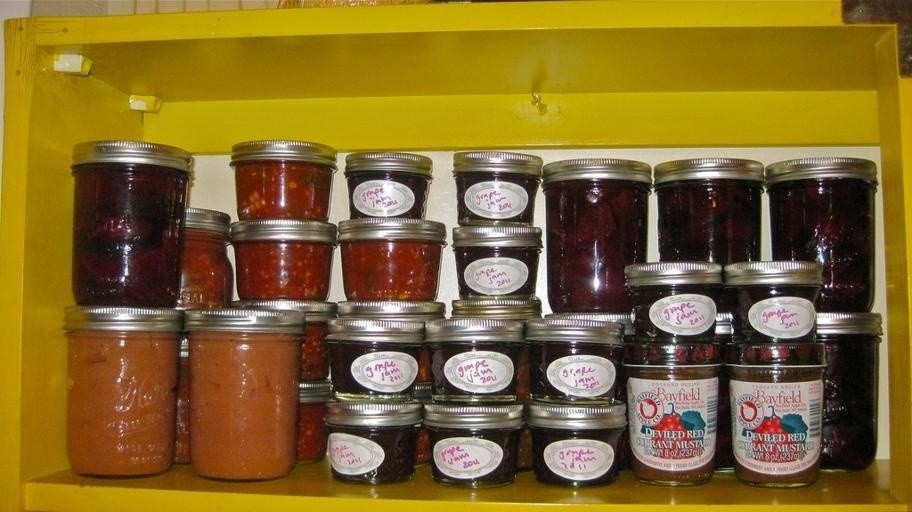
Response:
[655,155,764,263]
[452,225,542,300]
[527,319,625,405]
[725,261,823,345]
[232,219,335,299]
[229,139,338,222]
[323,398,425,484]
[61,305,184,478]
[338,220,445,302]
[72,141,191,312]
[725,346,827,488]
[326,317,421,400]
[816,314,882,473]
[531,404,629,489]
[344,153,432,219]
[624,261,722,340]
[542,158,649,316]
[452,152,544,226]
[765,156,877,312]
[188,308,305,476]
[622,344,729,487]
[241,299,335,382]
[430,318,525,403]
[296,381,327,464]
[425,402,524,490]
[181,206,233,308]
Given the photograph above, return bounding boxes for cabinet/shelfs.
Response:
[2,1,912,512]
